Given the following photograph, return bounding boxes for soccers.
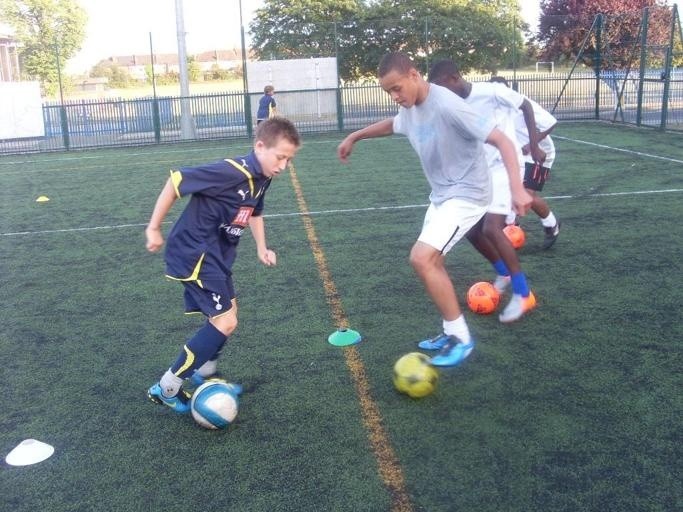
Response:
[467,281,499,314]
[191,382,239,429]
[393,352,438,397]
[503,225,524,249]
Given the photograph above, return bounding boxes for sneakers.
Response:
[494,274,511,290]
[188,371,241,393]
[543,218,560,248]
[146,381,190,412]
[499,288,537,323]
[418,332,449,350]
[432,334,474,367]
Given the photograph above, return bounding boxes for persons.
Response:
[337,52,532,368]
[256,85,275,127]
[143,117,302,412]
[485,76,561,251]
[426,60,546,323]
